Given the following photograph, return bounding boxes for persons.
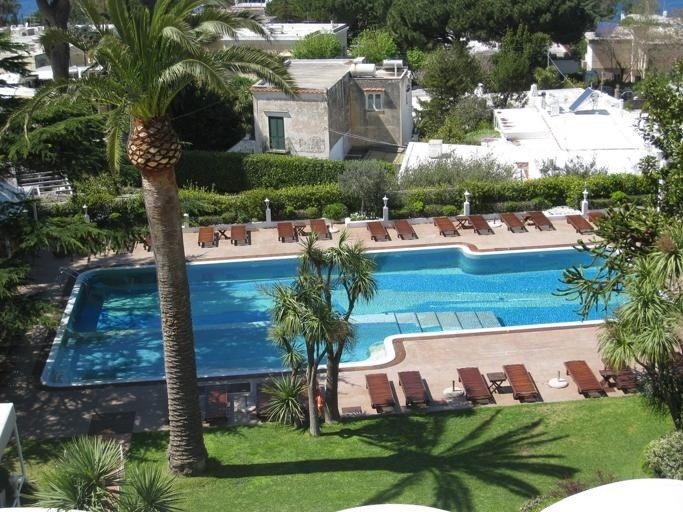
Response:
[472,83,483,98]
[610,82,621,109]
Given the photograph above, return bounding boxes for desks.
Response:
[294,222,306,235]
[455,216,469,228]
[383,222,395,231]
[216,223,230,240]
[519,212,531,223]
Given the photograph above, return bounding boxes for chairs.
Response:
[589,212,607,224]
[198,226,219,248]
[503,364,538,403]
[566,215,595,235]
[457,367,493,406]
[367,221,391,242]
[144,230,152,250]
[206,378,323,424]
[528,212,557,232]
[310,219,332,240]
[564,360,604,398]
[601,357,640,393]
[433,217,460,237]
[398,371,430,408]
[468,216,495,234]
[365,373,395,413]
[230,225,248,245]
[393,219,419,239]
[500,212,529,234]
[277,221,294,243]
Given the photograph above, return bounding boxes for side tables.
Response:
[487,372,506,394]
[599,370,616,387]
